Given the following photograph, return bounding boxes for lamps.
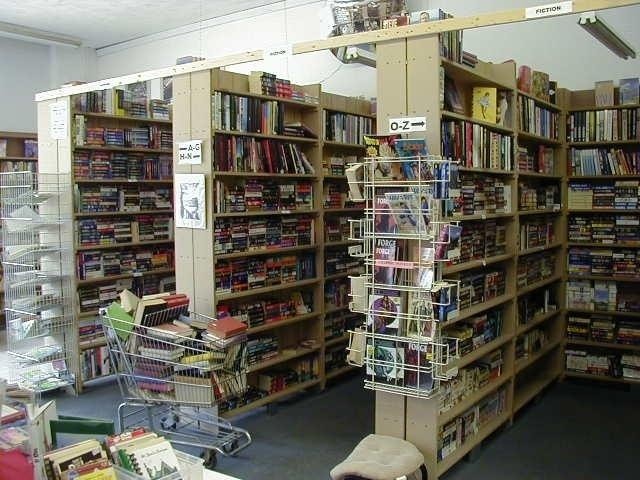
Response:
[576,13,640,60]
[344,48,383,67]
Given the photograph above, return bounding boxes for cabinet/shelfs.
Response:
[405,56,512,477]
[344,156,464,400]
[513,87,562,414]
[0,170,77,403]
[563,104,639,386]
[191,89,323,420]
[55,110,175,392]
[1,131,40,346]
[321,106,376,381]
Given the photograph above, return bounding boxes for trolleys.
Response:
[98,293,252,471]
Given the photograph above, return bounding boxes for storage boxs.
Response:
[113,450,205,480]
[112,468,206,480]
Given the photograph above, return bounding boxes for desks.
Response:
[204,468,241,480]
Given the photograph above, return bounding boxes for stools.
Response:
[330,433,427,480]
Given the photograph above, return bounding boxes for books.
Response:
[0,140,75,399]
[564,76,640,381]
[409,9,478,69]
[516,65,563,361]
[0,377,183,480]
[345,77,511,463]
[63,80,176,381]
[105,288,247,406]
[211,71,320,413]
[323,110,376,374]
